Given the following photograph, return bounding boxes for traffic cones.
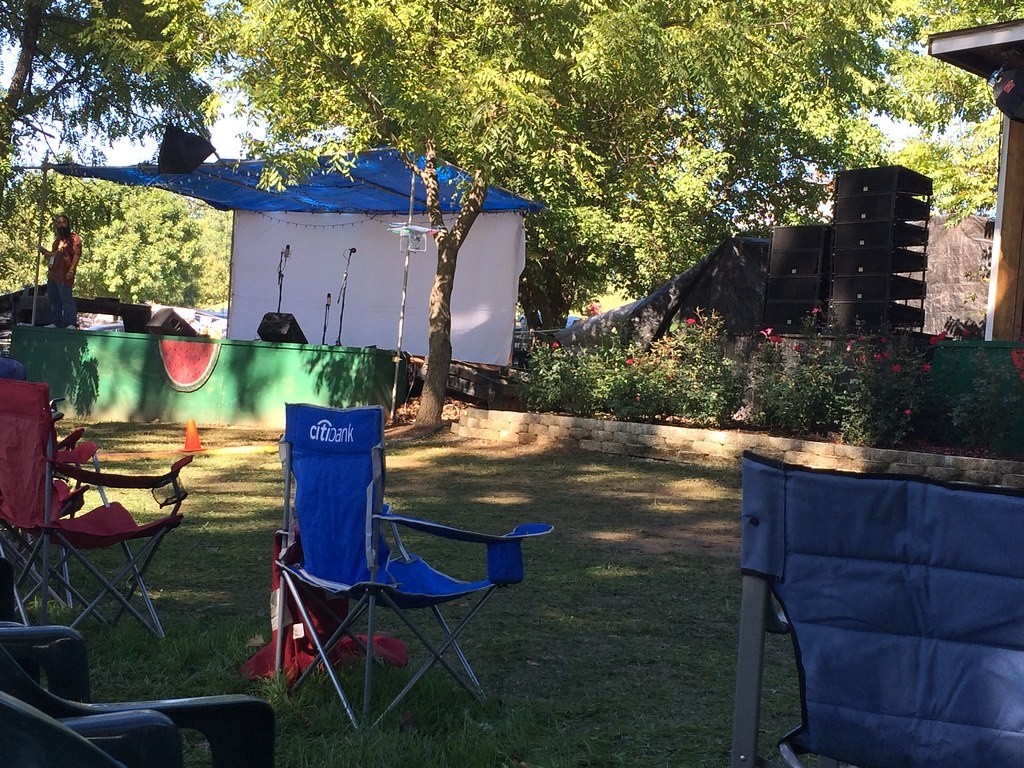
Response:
[178,419,207,453]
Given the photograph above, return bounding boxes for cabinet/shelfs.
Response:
[764,226,835,342]
[826,166,933,344]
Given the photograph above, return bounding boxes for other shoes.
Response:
[66,325,77,330]
[44,324,57,329]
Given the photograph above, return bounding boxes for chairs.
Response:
[0,357,275,768]
[730,451,1024,768]
[276,403,554,737]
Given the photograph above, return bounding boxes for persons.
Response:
[39,215,83,330]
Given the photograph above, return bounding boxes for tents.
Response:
[29,146,543,423]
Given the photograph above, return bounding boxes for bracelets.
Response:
[69,269,75,274]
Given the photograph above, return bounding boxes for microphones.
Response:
[349,248,356,253]
[284,244,290,261]
[327,293,331,310]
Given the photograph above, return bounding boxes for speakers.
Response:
[146,307,198,337]
[724,165,932,336]
[257,313,309,344]
